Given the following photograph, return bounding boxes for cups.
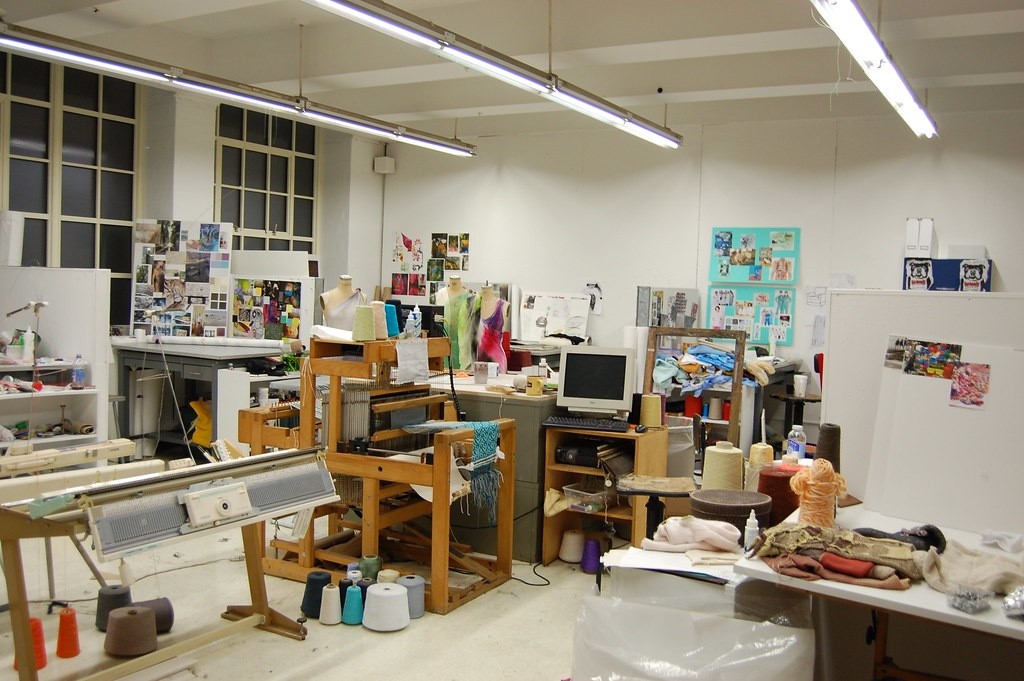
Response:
[473,362,489,384]
[526,376,544,396]
[6,345,22,362]
[794,375,808,397]
[488,362,499,378]
[550,371,560,382]
[521,365,539,375]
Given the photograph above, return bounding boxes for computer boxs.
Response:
[401,305,444,337]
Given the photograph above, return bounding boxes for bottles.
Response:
[744,509,759,549]
[22,327,35,362]
[279,391,301,427]
[413,302,422,338]
[539,358,548,379]
[406,310,415,338]
[787,425,806,459]
[72,354,84,390]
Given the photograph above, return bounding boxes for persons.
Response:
[431,275,475,372]
[159,221,179,251]
[712,306,722,330]
[152,260,165,293]
[320,275,368,331]
[465,282,510,375]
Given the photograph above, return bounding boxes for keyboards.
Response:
[542,416,630,433]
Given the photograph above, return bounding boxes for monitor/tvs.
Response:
[556,345,636,414]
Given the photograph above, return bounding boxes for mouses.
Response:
[635,424,648,433]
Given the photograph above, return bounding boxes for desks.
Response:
[668,362,800,437]
[770,390,821,427]
[735,503,1024,681]
[510,343,560,368]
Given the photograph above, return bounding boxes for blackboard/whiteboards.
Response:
[821,289,1024,536]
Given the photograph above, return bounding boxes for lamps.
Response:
[0,21,478,158]
[810,0,939,138]
[304,0,683,149]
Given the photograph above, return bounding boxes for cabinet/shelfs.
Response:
[541,423,668,568]
[342,392,574,564]
[0,358,97,448]
[664,379,756,460]
[111,351,321,463]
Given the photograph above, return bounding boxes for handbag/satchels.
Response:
[183,397,213,447]
